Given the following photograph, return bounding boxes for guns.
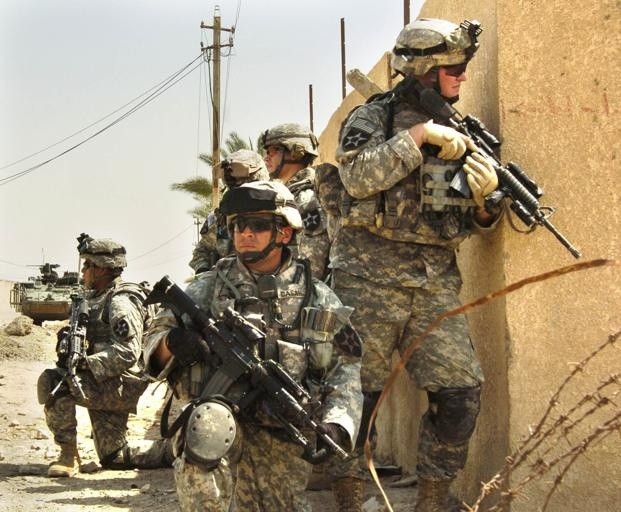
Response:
[143,276,350,463]
[50,294,90,401]
[392,73,582,261]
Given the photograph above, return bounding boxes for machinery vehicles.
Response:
[11,262,85,327]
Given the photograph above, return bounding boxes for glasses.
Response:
[229,217,272,232]
[443,65,466,77]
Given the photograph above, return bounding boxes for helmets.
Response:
[76,233,126,268]
[213,124,319,238]
[390,18,472,76]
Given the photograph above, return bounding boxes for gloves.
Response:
[425,122,480,159]
[303,422,343,465]
[464,150,498,195]
[164,327,211,360]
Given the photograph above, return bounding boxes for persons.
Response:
[260,123,330,282]
[324,18,505,511]
[37,239,174,478]
[140,180,364,511]
[187,148,270,273]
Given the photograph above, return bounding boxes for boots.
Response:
[48,444,81,477]
